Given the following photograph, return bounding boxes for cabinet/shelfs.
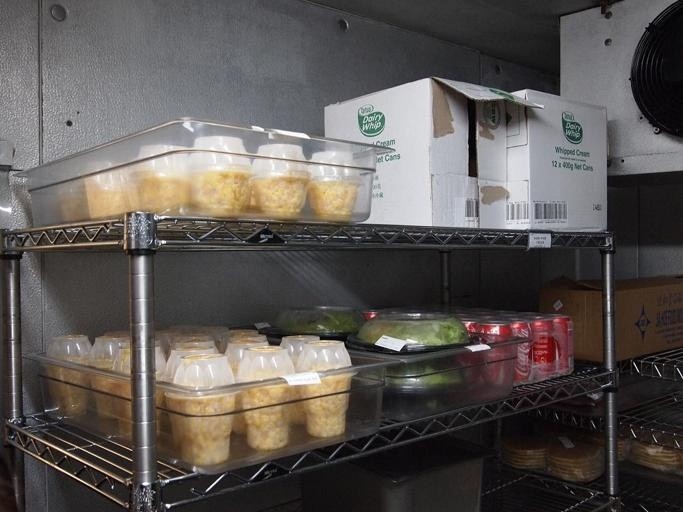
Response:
[498,350,682,512]
[0,211,618,512]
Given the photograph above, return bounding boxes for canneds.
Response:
[362,306,574,387]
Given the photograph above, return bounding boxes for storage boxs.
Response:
[537,275,681,364]
[321,76,546,229]
[260,335,533,423]
[23,349,403,476]
[509,89,608,233]
